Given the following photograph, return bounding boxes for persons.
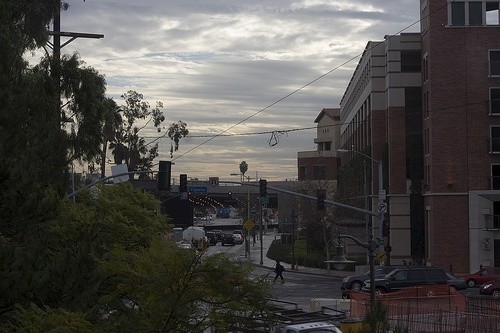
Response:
[273,259,284,282]
[377,245,391,265]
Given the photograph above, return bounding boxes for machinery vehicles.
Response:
[182,226,208,246]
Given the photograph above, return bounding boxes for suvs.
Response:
[206,230,224,245]
[360,268,447,298]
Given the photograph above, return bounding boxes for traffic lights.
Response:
[382,220,389,236]
[180,174,188,192]
[228,191,232,198]
[317,194,325,208]
[259,180,267,197]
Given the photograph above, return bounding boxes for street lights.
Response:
[335,148,384,244]
[291,209,298,270]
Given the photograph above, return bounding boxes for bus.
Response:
[165,228,183,242]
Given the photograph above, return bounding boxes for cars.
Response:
[445,272,465,291]
[479,279,500,298]
[342,266,400,291]
[222,230,244,246]
[464,267,500,287]
[179,244,191,249]
